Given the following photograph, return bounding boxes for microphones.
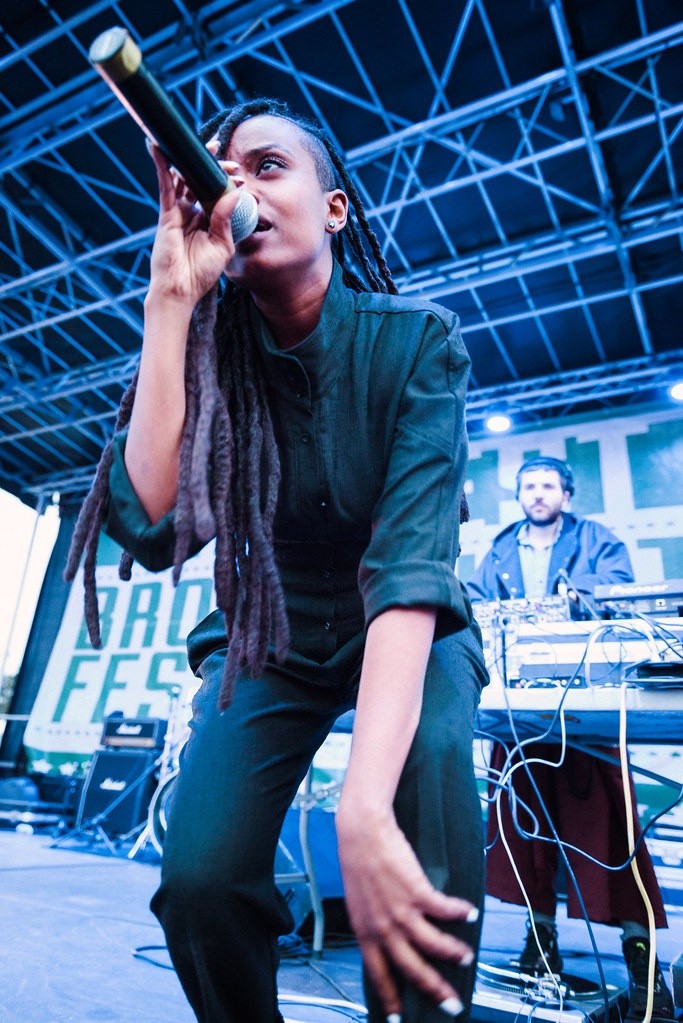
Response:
[91,25,259,242]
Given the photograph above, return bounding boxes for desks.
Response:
[273,685,683,961]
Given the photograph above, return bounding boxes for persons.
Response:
[463,457,676,1023]
[100,99,486,1023]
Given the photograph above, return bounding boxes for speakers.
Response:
[76,750,160,838]
[270,808,358,944]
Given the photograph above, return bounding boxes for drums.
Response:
[148,767,180,857]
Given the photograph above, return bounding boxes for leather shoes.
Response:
[519,922,563,973]
[624,937,673,1017]
